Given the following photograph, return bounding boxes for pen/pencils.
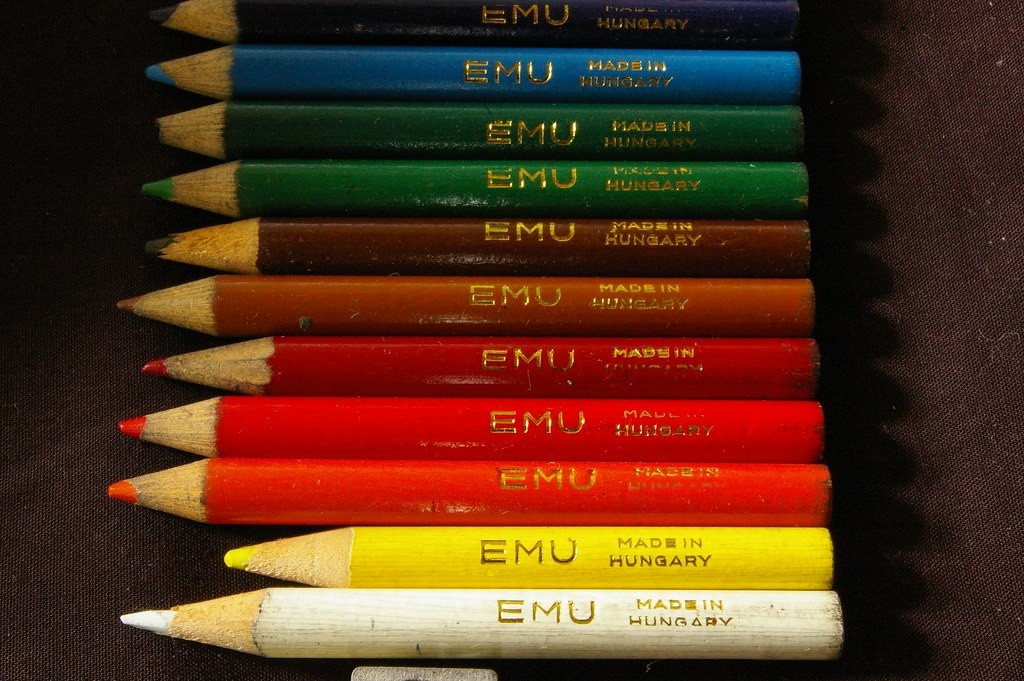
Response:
[144,43,802,105]
[142,335,820,398]
[118,395,824,462]
[156,100,804,162]
[119,588,845,659]
[223,526,835,589]
[141,159,809,219]
[144,216,812,278]
[148,1,801,50]
[117,274,815,336]
[109,457,832,526]
